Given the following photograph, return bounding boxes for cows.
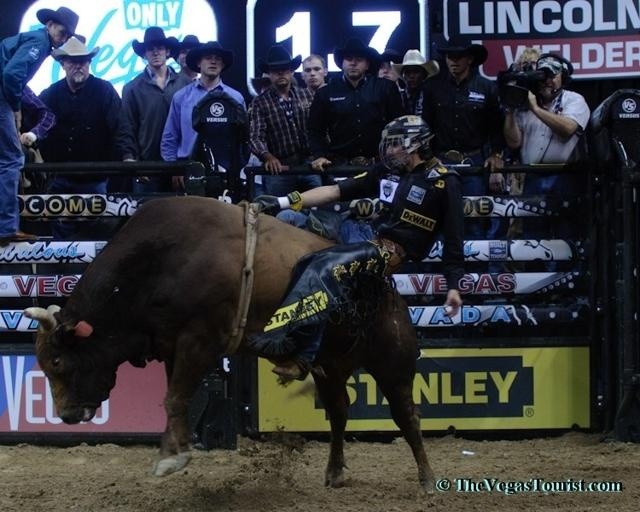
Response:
[21,195,439,498]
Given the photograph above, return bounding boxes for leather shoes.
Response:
[10,231,36,242]
[272,362,306,380]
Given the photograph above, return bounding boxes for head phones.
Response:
[536,54,569,85]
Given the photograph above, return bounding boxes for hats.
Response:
[186,42,234,74]
[538,57,564,76]
[173,34,206,63]
[133,28,179,60]
[50,37,100,62]
[259,46,302,74]
[334,39,442,84]
[436,36,489,68]
[252,76,273,97]
[37,8,86,45]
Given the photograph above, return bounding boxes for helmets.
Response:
[379,114,436,171]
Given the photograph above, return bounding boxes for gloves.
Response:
[253,191,303,217]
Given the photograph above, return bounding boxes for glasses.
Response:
[304,67,321,72]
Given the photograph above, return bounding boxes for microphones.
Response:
[551,81,570,100]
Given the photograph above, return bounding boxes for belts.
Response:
[345,156,379,166]
[439,149,480,161]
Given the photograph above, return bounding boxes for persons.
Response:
[33,35,123,242]
[114,25,195,186]
[1,6,86,244]
[159,33,590,334]
[252,114,466,382]
[14,83,55,150]
